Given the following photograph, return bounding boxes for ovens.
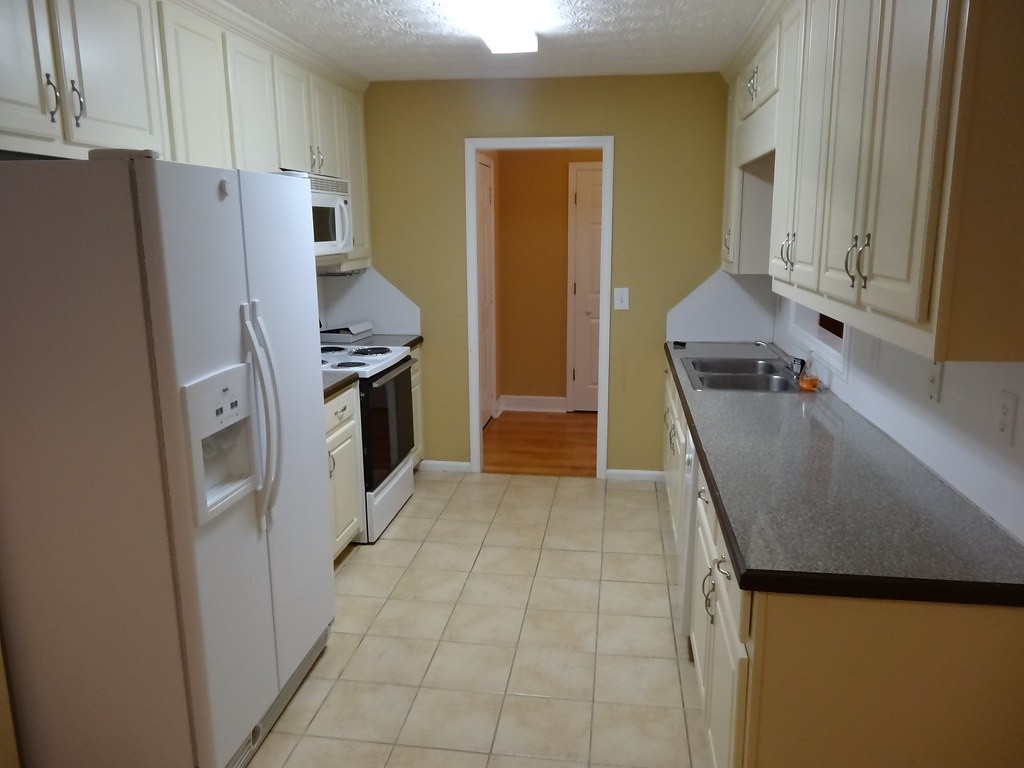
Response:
[351,355,418,543]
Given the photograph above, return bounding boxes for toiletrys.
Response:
[799,350,819,390]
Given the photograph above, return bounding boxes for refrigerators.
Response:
[0,149,335,768]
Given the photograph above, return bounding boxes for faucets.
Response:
[754,340,806,375]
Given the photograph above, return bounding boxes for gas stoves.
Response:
[321,345,410,378]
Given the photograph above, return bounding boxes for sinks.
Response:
[688,375,827,394]
[681,357,780,374]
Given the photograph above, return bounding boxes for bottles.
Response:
[798,349,819,390]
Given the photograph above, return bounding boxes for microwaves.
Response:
[309,174,354,255]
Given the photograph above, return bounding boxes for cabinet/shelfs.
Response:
[738,24,781,121]
[324,380,366,553]
[410,343,425,468]
[660,375,691,538]
[720,82,773,276]
[687,467,753,768]
[338,86,373,272]
[819,0,964,321]
[158,0,281,174]
[0,0,171,161]
[769,0,842,292]
[273,54,343,178]
